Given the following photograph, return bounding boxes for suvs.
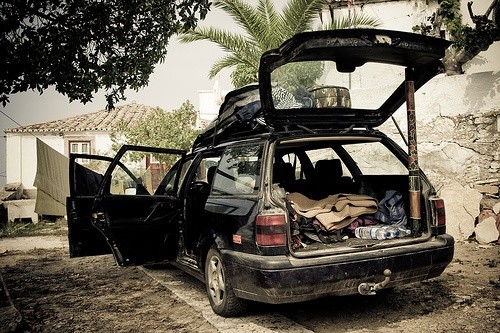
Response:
[65,28,455,317]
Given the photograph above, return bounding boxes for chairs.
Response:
[306,156,352,198]
[270,157,297,195]
[200,164,235,202]
